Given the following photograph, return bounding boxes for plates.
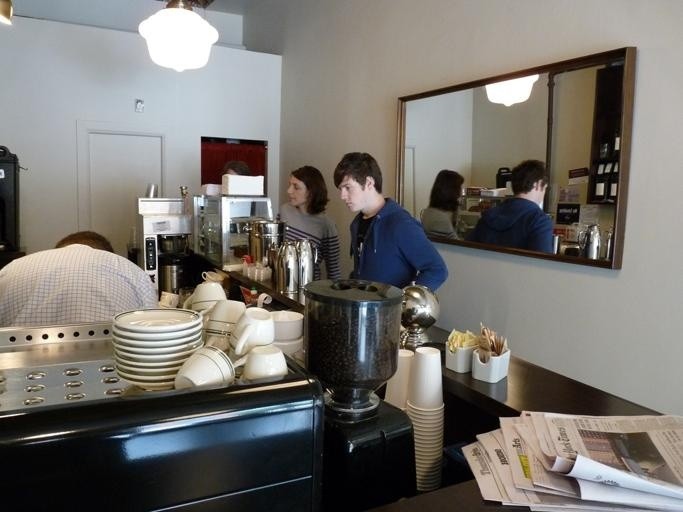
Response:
[112,307,204,392]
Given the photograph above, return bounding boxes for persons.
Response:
[332,151,449,294]
[466,158,554,253]
[218,160,250,176]
[421,168,465,241]
[1,229,158,327]
[275,165,342,287]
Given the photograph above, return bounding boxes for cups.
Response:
[384,347,445,492]
[174,344,236,392]
[238,343,288,386]
[161,291,180,307]
[182,281,305,362]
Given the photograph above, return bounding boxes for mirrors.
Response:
[396,46,636,271]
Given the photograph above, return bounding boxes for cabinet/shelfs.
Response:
[188,195,273,270]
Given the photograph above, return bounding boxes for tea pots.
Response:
[276,239,299,294]
[296,238,318,289]
[574,222,602,260]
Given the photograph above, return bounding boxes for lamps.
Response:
[481,76,539,107]
[137,0,218,72]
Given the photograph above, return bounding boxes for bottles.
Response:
[592,133,622,200]
